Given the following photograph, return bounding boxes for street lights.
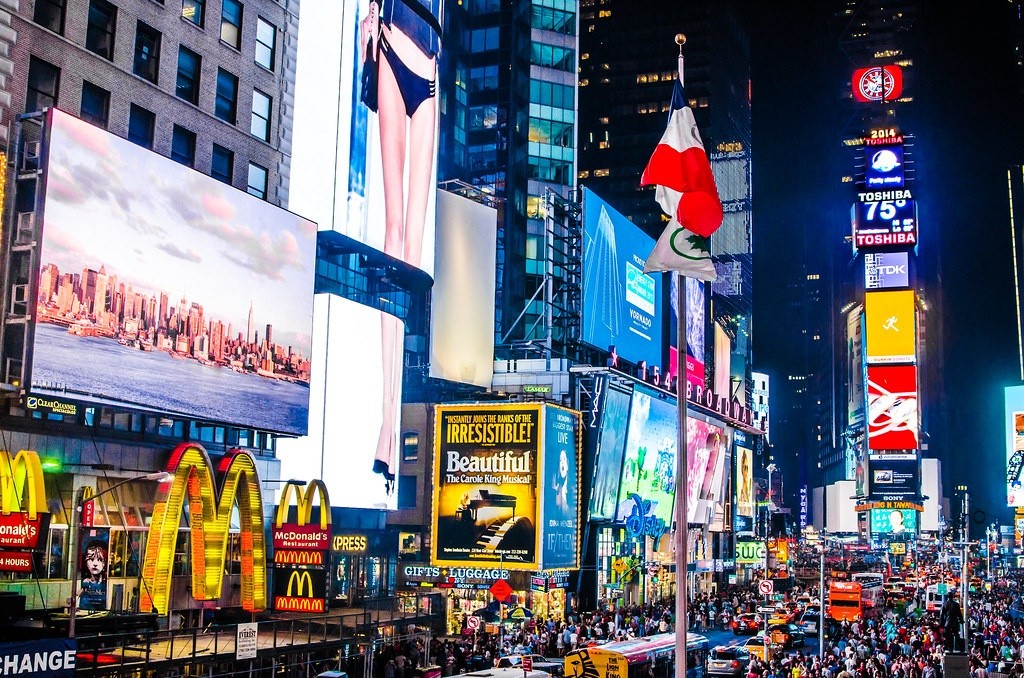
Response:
[66,472,169,638]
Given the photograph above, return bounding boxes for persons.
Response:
[740,450,750,502]
[374,311,401,480]
[557,450,568,513]
[889,510,906,532]
[286,540,1024,678]
[360,0,440,268]
[82,540,106,584]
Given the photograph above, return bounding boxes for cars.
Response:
[733,596,829,642]
[707,645,752,677]
[859,561,994,619]
[743,635,782,663]
[495,654,551,670]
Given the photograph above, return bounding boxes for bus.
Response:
[828,580,873,625]
[562,632,717,678]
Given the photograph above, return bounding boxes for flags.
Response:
[640,72,724,281]
